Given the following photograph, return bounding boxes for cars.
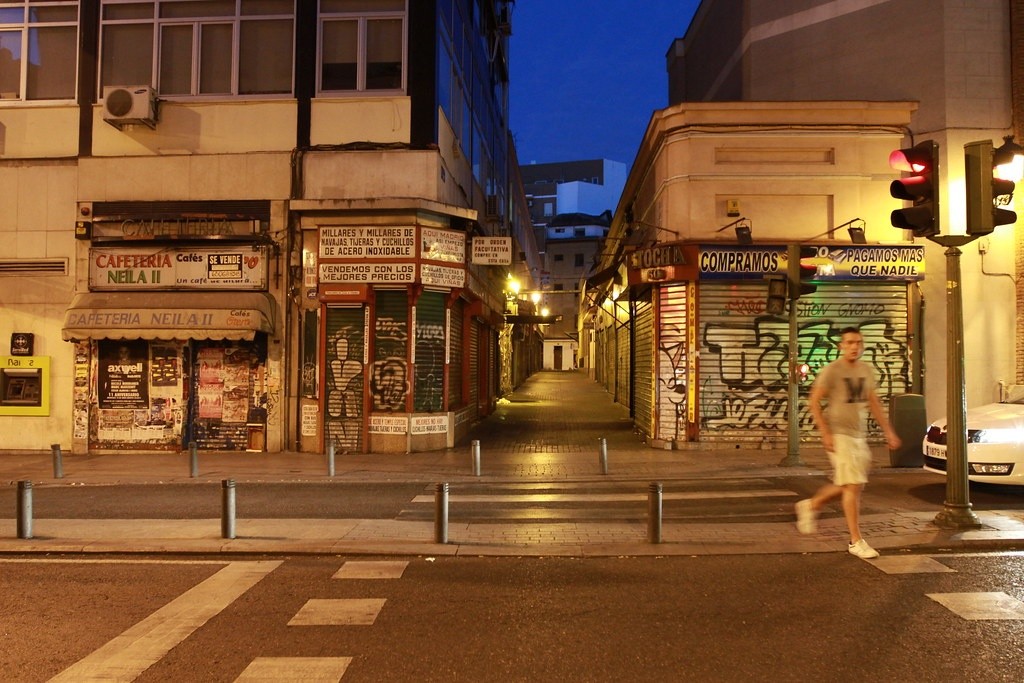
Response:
[922,382,1024,488]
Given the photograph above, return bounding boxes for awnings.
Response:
[586,257,629,328]
[62,292,276,340]
[507,308,563,329]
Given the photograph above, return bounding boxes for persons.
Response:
[119,345,130,360]
[795,327,902,559]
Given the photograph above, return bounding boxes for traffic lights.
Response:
[967,141,1016,237]
[889,140,940,237]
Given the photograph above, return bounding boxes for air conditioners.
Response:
[103,85,157,126]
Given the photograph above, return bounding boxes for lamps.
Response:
[807,218,868,245]
[717,216,753,245]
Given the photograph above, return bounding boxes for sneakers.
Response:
[794,499,819,535]
[847,540,880,558]
